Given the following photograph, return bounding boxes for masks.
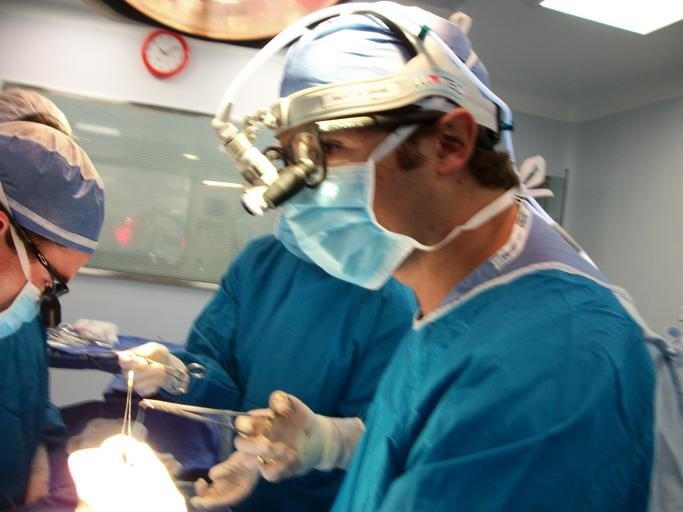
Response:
[272,159,417,292]
[1,228,41,340]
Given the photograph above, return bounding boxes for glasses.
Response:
[7,207,70,300]
[263,129,332,188]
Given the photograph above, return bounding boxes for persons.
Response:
[234,0,656,511]
[1,88,106,512]
[114,214,416,512]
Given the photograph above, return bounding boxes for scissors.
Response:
[111,349,208,395]
[46,323,112,349]
[142,390,294,466]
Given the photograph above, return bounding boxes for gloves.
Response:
[189,450,260,511]
[234,389,366,483]
[113,340,188,398]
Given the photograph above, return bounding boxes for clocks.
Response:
[142,31,188,76]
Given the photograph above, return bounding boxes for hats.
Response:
[278,1,490,139]
[0,87,74,139]
[0,121,106,252]
[212,14,308,183]
